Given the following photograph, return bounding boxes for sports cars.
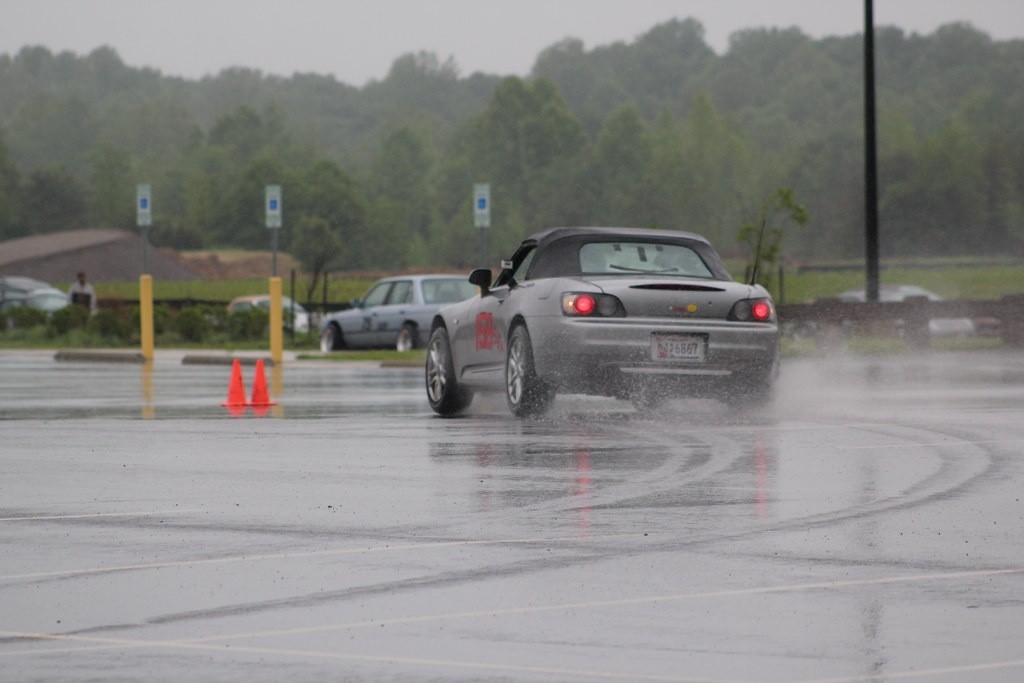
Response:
[424,224,780,422]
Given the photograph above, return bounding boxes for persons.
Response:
[68,271,96,313]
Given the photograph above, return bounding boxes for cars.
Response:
[226,295,309,336]
[814,282,978,340]
[0,275,71,317]
[318,274,477,354]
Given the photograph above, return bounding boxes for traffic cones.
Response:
[250,359,278,408]
[219,359,250,406]
